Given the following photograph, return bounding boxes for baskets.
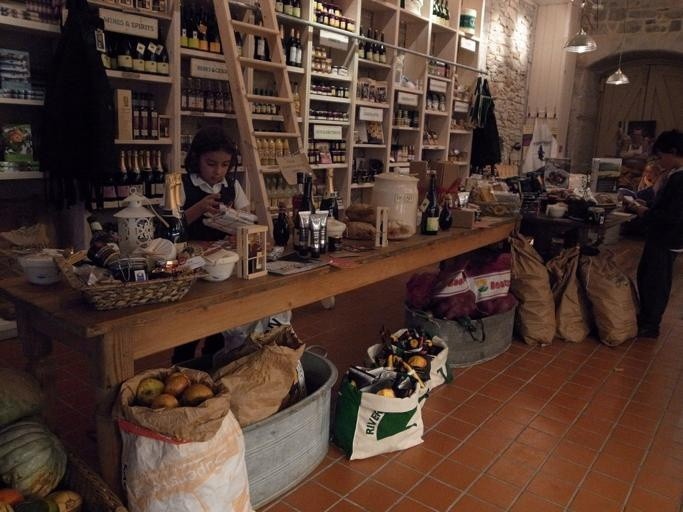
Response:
[70,265,213,314]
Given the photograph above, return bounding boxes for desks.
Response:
[521,207,616,264]
[0,216,522,504]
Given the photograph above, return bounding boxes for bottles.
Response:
[328,4,335,14]
[215,81,223,112]
[236,31,243,57]
[302,176,314,214]
[313,45,350,75]
[439,93,446,111]
[274,202,289,247]
[440,0,446,23]
[332,141,337,162]
[287,29,296,65]
[87,214,117,252]
[149,94,158,140]
[365,28,374,59]
[180,6,188,47]
[335,8,341,16]
[254,89,260,113]
[439,194,453,233]
[293,0,301,17]
[151,151,157,170]
[311,83,332,96]
[210,16,221,53]
[352,20,355,31]
[427,92,432,110]
[193,79,203,111]
[292,172,306,250]
[346,18,352,30]
[130,151,143,197]
[380,32,386,63]
[139,150,145,170]
[283,0,292,15]
[340,17,346,29]
[317,1,324,12]
[158,174,182,242]
[260,89,266,113]
[344,88,349,98]
[276,137,283,157]
[261,138,269,165]
[256,20,268,60]
[173,175,184,224]
[329,14,334,25]
[268,137,276,165]
[187,79,195,110]
[336,141,341,163]
[275,0,283,13]
[279,24,286,64]
[116,150,129,200]
[256,138,261,158]
[433,93,439,110]
[359,26,365,58]
[266,89,271,114]
[342,142,347,162]
[412,146,416,161]
[373,30,380,61]
[323,13,330,25]
[117,35,132,70]
[134,36,144,72]
[283,138,291,159]
[334,16,341,27]
[189,8,199,49]
[205,80,214,111]
[155,150,166,196]
[408,145,411,161]
[393,109,419,127]
[338,87,344,97]
[310,141,315,164]
[182,78,187,109]
[447,1,449,25]
[422,174,438,235]
[158,41,170,76]
[224,81,232,114]
[144,151,155,196]
[320,169,338,221]
[317,11,324,23]
[132,90,139,139]
[181,134,193,167]
[139,92,149,139]
[433,0,439,22]
[309,110,349,121]
[271,90,277,114]
[296,30,303,67]
[201,8,209,51]
[145,40,157,74]
[323,2,328,13]
[316,149,319,164]
[332,86,338,96]
[126,151,132,172]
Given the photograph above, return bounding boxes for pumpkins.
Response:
[0,489,23,503]
[1,421,65,497]
[1,367,39,424]
[56,493,82,512]
[16,498,58,512]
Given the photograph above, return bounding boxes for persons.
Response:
[619,130,683,339]
[638,163,661,190]
[171,128,252,364]
[620,128,647,159]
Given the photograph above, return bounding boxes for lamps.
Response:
[606,1,630,85]
[563,0,597,53]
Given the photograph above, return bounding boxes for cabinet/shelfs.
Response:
[177,0,251,214]
[449,0,484,177]
[421,0,460,172]
[387,0,432,175]
[349,0,399,208]
[1,0,71,342]
[83,0,178,211]
[250,0,312,222]
[307,0,360,211]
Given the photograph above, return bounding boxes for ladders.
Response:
[214,0,305,247]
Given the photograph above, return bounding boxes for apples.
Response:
[183,383,213,405]
[137,377,164,404]
[407,355,428,367]
[166,375,190,394]
[152,394,176,409]
[409,339,419,349]
[378,389,394,400]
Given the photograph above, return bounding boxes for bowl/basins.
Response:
[548,206,568,218]
[17,252,66,285]
[201,251,240,282]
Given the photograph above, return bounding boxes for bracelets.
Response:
[182,211,189,226]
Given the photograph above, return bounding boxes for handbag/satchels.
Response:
[332,367,424,461]
[368,338,453,394]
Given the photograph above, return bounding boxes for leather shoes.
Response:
[638,329,659,339]
